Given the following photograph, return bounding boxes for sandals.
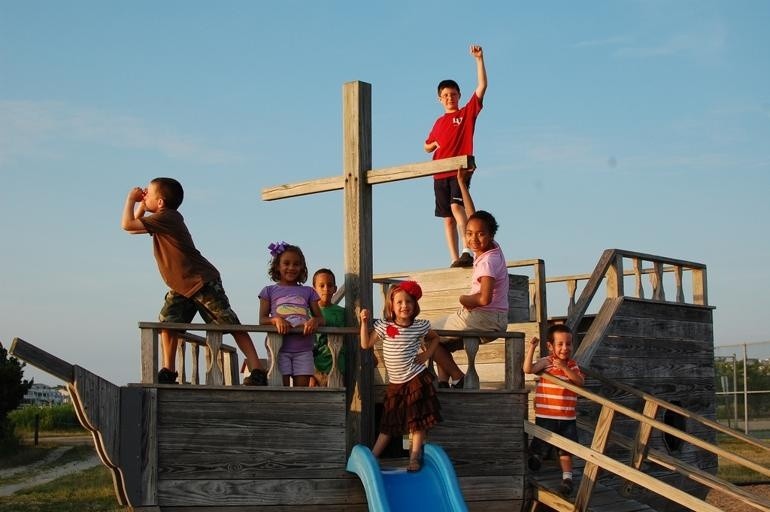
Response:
[529,454,541,471]
[438,375,465,389]
[406,451,423,472]
[561,477,575,494]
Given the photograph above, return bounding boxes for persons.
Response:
[427,163,508,388]
[123,177,267,386]
[258,243,325,386]
[525,324,585,493]
[306,268,345,386]
[359,281,440,472]
[426,45,488,268]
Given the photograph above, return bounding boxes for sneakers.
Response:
[241,370,268,385]
[158,368,178,383]
